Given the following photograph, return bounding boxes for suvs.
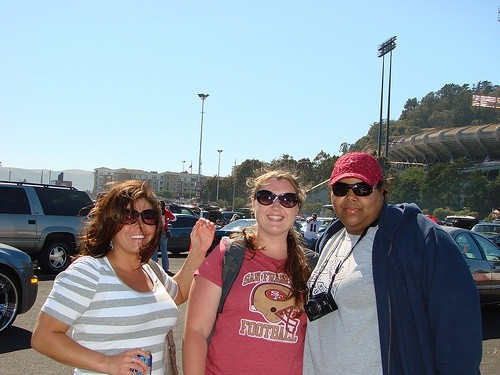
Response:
[0,180,97,273]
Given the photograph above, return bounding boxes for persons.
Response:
[182,167,311,375]
[303,152,482,375]
[30,178,216,375]
[492,209,500,223]
[151,200,178,277]
[304,213,331,240]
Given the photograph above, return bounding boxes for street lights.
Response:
[181,160,186,172]
[232,157,239,212]
[377,34,398,159]
[197,93,210,198]
[216,149,224,203]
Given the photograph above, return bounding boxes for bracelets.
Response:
[184,262,196,273]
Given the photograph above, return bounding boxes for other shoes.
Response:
[166,271,175,276]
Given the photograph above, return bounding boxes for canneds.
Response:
[131,349,152,375]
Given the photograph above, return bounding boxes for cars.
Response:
[439,215,500,248]
[160,203,342,255]
[438,225,500,316]
[0,240,39,332]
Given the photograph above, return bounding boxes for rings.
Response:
[131,356,138,363]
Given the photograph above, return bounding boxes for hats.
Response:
[329,152,384,186]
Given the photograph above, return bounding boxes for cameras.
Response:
[304,292,339,322]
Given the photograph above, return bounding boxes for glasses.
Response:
[119,209,158,226]
[255,190,299,208]
[331,182,373,197]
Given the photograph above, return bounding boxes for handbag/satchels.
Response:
[166,230,172,240]
